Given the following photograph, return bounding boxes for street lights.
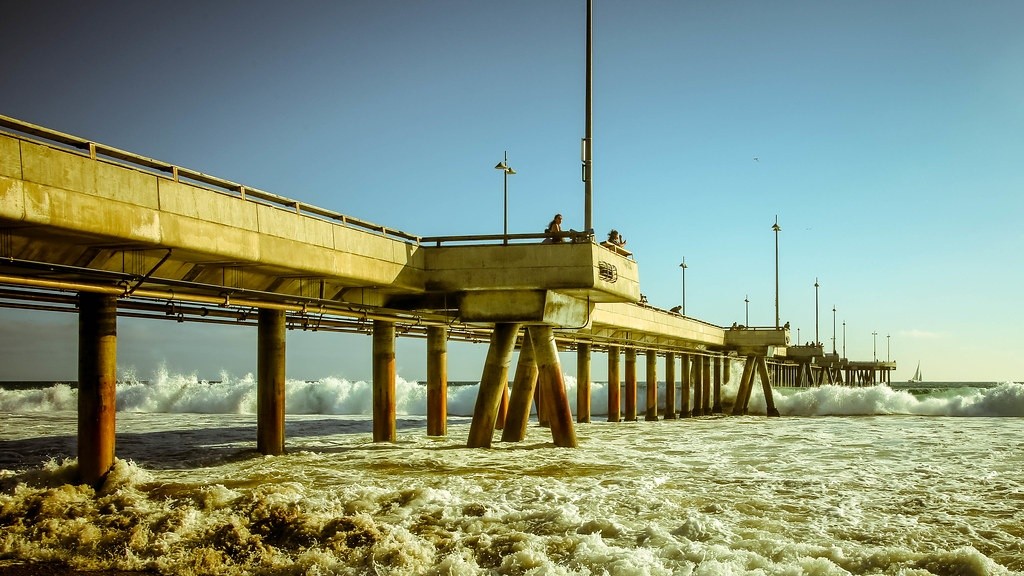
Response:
[872,330,877,362]
[679,257,688,319]
[833,306,837,353]
[842,320,846,358]
[744,294,750,329]
[887,334,890,362]
[814,277,820,348]
[772,215,782,330]
[495,151,516,244]
[798,328,800,346]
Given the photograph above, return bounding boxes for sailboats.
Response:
[908,362,925,383]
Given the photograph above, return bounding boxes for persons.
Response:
[607,229,626,253]
[668,306,682,318]
[542,229,552,242]
[549,214,566,243]
[638,294,648,308]
[781,322,790,331]
[806,341,824,349]
[730,322,744,331]
